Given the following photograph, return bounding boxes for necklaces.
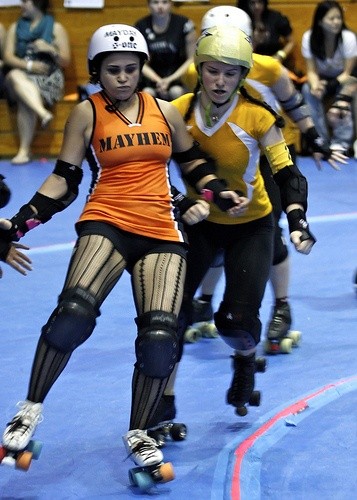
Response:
[212,116,219,120]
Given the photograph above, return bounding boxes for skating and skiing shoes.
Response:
[184,298,219,343]
[145,394,187,449]
[0,400,43,472]
[262,306,303,353]
[121,428,176,494]
[227,351,267,416]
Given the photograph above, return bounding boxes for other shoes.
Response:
[10,153,32,164]
[40,113,54,128]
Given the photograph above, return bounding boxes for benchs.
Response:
[1,93,79,158]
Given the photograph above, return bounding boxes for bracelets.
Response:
[51,51,58,58]
[27,61,33,72]
[276,50,286,59]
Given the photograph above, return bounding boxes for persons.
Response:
[4,0,72,164]
[0,23,249,492]
[237,0,357,159]
[147,27,318,449]
[134,0,194,100]
[178,6,350,356]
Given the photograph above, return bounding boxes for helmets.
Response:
[88,24,150,76]
[194,26,252,88]
[201,6,252,40]
